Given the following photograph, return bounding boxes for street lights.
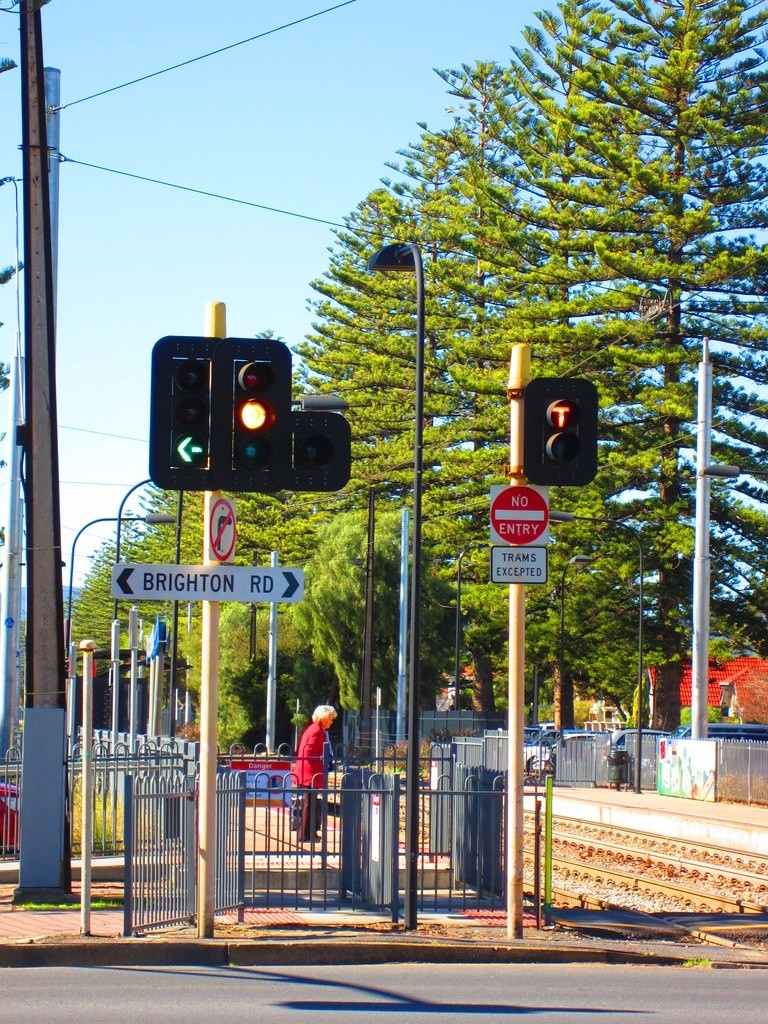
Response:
[67,515,176,663]
[558,556,592,678]
[547,510,644,797]
[455,542,492,711]
[365,237,426,931]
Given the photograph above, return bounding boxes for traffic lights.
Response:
[222,334,298,497]
[149,335,223,492]
[518,378,600,488]
[294,406,355,492]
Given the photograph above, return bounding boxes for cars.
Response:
[522,724,768,780]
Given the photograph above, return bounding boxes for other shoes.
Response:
[307,833,321,843]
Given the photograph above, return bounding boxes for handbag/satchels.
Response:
[289,796,323,831]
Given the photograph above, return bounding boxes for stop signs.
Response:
[490,486,553,543]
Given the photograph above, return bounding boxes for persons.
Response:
[291,704,337,841]
